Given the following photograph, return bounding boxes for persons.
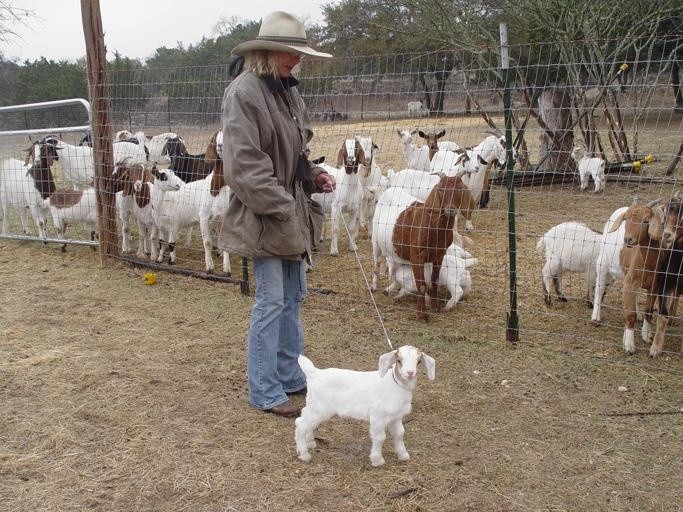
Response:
[219,11,336,418]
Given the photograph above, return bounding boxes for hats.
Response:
[229,10,333,63]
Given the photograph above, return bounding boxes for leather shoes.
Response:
[267,399,303,419]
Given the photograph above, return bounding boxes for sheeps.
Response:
[0,126,232,280]
[570,147,607,194]
[592,190,683,357]
[294,343,437,468]
[357,136,385,238]
[534,220,615,312]
[406,101,432,120]
[307,137,366,257]
[366,126,518,325]
